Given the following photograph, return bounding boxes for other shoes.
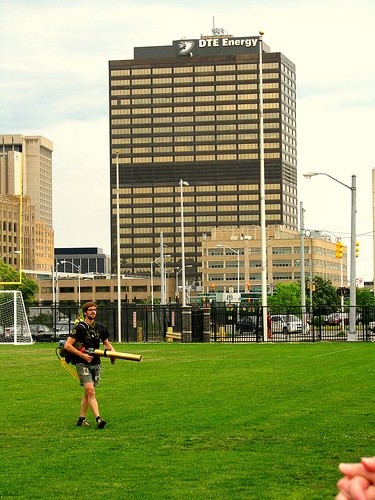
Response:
[76,420,89,427]
[97,419,106,428]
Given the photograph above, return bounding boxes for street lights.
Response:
[151,255,171,341]
[178,178,190,303]
[60,261,81,312]
[215,245,240,322]
[189,281,201,304]
[303,171,359,342]
[314,229,344,286]
[175,265,193,302]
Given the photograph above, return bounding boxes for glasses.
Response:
[87,309,97,311]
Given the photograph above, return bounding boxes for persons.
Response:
[334,455,375,500]
[63,302,116,428]
[74,314,84,327]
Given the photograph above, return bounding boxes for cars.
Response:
[235,315,263,334]
[23,324,54,343]
[308,312,349,325]
[270,314,309,335]
[52,321,77,342]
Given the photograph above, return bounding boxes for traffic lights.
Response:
[355,241,360,258]
[211,283,214,289]
[336,288,341,296]
[248,283,250,290]
[344,287,350,297]
[335,242,343,259]
